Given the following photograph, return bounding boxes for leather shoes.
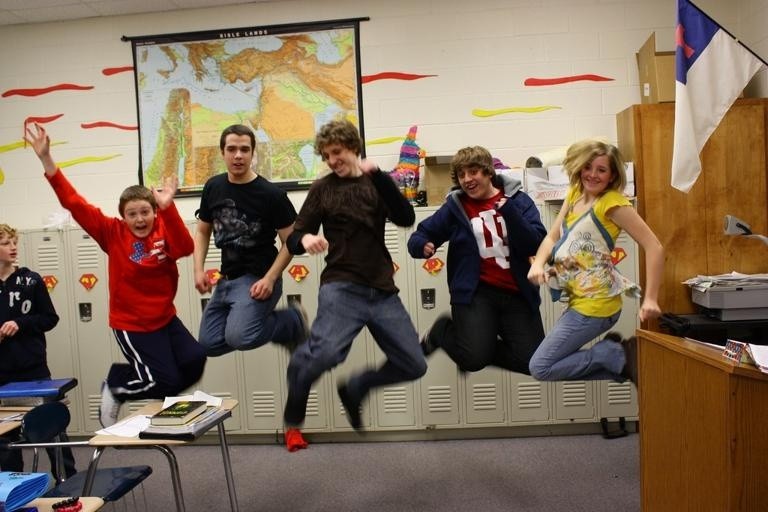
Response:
[623,335,638,389]
[603,331,628,384]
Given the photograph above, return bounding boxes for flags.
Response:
[671,1,768,194]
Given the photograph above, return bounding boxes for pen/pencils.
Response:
[0,413,23,422]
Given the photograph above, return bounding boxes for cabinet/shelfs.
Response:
[635,327,768,512]
[614,98,768,331]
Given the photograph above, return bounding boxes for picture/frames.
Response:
[120,15,372,193]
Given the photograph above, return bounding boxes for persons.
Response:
[282,119,427,431]
[527,142,664,388]
[194,125,309,356]
[0,222,77,485]
[407,147,547,375]
[22,121,206,428]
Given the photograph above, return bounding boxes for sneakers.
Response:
[291,300,310,340]
[98,379,119,429]
[337,382,370,435]
[420,312,450,357]
[285,429,308,452]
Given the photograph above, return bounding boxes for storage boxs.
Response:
[423,164,459,205]
[13,198,642,445]
[493,168,524,192]
[525,167,549,201]
[546,161,636,198]
[636,26,680,103]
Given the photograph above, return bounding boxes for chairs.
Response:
[22,401,152,511]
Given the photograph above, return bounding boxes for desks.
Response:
[17,495,105,512]
[0,405,34,441]
[81,396,240,512]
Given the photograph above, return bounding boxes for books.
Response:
[149,401,220,430]
